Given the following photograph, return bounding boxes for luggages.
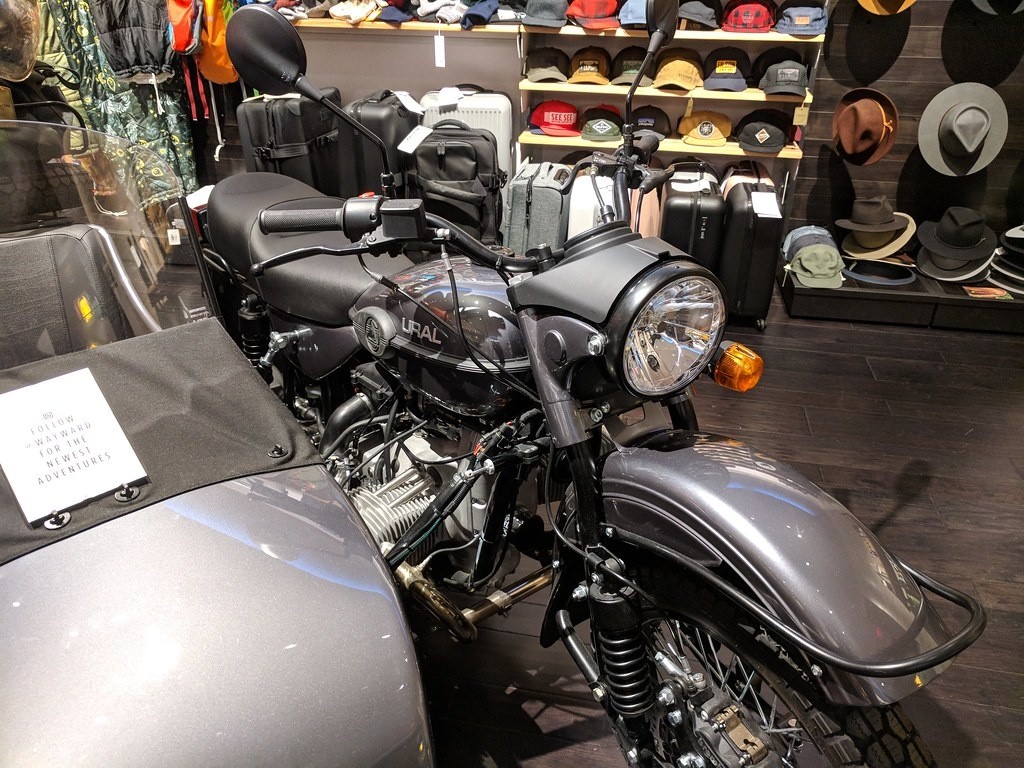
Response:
[567,152,633,241]
[628,153,665,240]
[717,160,782,329]
[658,154,725,276]
[418,84,513,239]
[341,88,416,200]
[405,118,509,248]
[235,89,340,197]
[500,161,572,257]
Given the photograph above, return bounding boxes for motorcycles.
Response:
[188,0,993,768]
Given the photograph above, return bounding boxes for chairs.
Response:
[0,221,132,370]
[0,141,58,229]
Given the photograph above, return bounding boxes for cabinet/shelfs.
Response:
[517,26,826,242]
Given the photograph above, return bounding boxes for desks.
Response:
[292,16,523,55]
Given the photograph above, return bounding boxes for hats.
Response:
[520,0,829,154]
[970,0,1024,14]
[833,86,899,166]
[917,81,1009,177]
[858,0,916,15]
[781,194,1024,294]
